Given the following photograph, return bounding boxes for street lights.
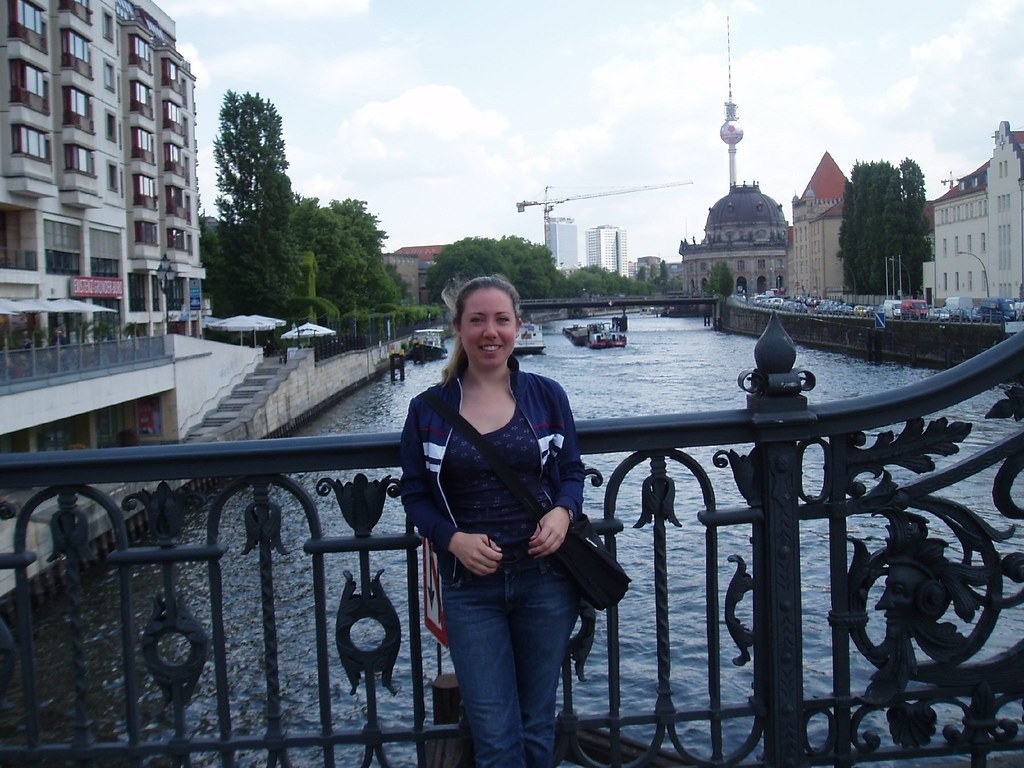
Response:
[957,251,990,298]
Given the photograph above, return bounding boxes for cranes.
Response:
[941,171,962,190]
[515,182,694,252]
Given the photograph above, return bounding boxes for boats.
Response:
[406,328,448,360]
[640,307,655,315]
[513,322,544,355]
[562,324,627,350]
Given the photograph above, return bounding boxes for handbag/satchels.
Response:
[558,514,632,612]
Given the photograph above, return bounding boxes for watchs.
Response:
[565,509,575,524]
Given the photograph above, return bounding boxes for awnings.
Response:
[16,298,119,315]
[0,298,20,316]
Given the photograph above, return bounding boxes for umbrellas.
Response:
[207,315,276,331]
[247,314,287,327]
[200,315,222,328]
[281,322,337,339]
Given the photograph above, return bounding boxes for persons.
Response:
[264,338,275,358]
[397,277,630,768]
[18,331,35,376]
[49,326,68,373]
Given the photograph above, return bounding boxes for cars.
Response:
[750,286,1024,323]
